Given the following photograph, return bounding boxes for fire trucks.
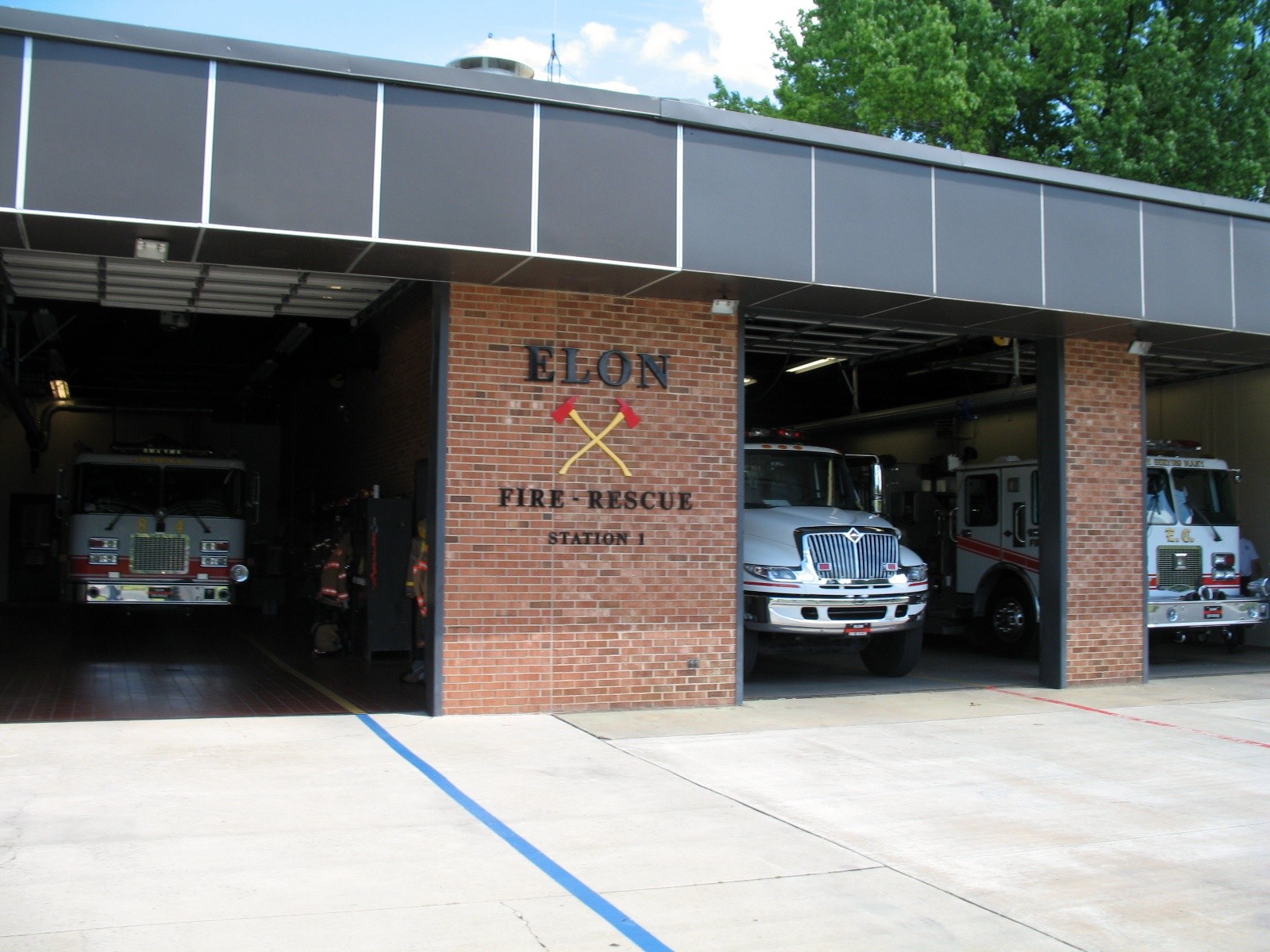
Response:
[745,425,930,678]
[55,442,265,647]
[889,438,1270,657]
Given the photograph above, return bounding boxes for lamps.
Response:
[49,378,70,400]
[34,309,60,345]
[710,291,739,315]
[784,356,847,374]
[1125,335,1153,355]
[136,239,170,262]
